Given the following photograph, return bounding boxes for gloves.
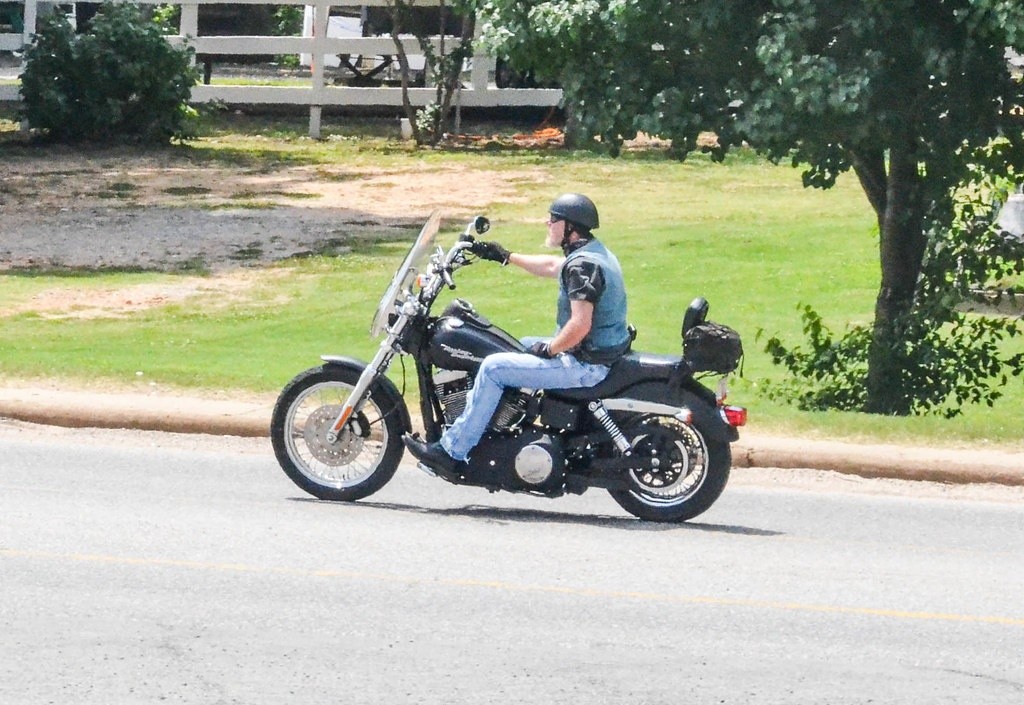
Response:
[528,341,553,360]
[476,240,512,267]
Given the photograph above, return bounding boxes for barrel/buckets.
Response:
[401,118,413,139]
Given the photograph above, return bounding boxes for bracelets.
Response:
[547,341,557,357]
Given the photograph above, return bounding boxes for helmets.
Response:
[546,192,600,228]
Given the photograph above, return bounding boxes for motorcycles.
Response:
[268,208,750,521]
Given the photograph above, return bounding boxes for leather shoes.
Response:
[400,431,461,474]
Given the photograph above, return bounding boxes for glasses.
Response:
[550,212,564,223]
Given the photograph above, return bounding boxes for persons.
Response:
[404,193,631,476]
